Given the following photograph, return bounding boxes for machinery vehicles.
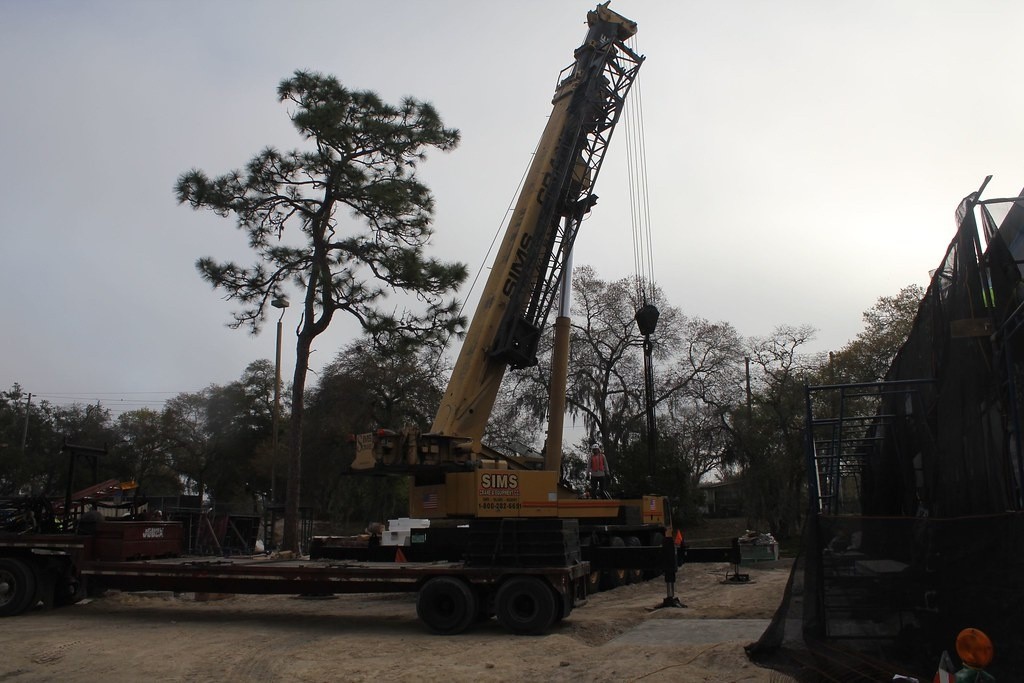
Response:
[305,2,677,636]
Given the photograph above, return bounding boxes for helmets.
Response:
[591,444,599,448]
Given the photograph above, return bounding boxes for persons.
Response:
[586,443,610,500]
